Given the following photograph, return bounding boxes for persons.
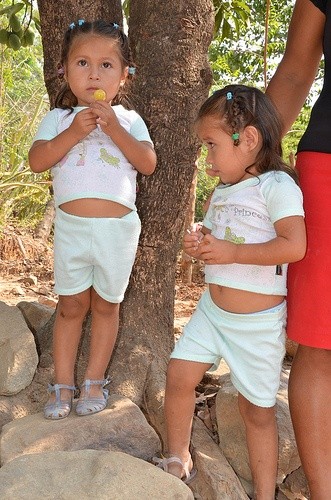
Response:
[203,0,331,500]
[28,19,157,420]
[153,84,306,500]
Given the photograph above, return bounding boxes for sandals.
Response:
[44,383,76,420]
[152,452,198,483]
[76,375,110,415]
